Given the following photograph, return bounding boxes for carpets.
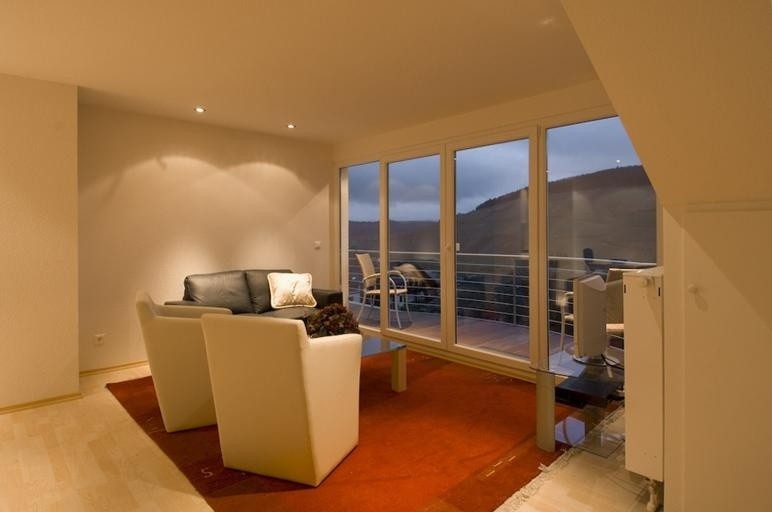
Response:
[104,349,620,512]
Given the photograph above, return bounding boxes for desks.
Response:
[527,343,624,453]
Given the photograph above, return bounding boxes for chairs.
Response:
[558,267,632,379]
[134,287,235,436]
[354,252,413,330]
[198,309,364,490]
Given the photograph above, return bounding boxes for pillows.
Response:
[265,270,317,309]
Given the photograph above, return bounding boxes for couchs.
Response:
[162,269,345,336]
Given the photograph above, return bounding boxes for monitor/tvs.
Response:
[573,274,620,366]
